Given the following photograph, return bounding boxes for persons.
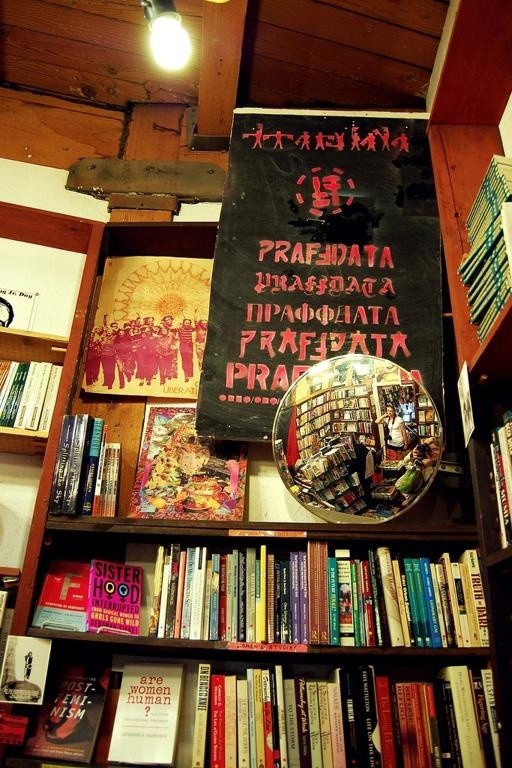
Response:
[85,313,209,390]
[374,405,409,460]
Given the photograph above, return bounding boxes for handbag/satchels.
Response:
[393,463,425,494]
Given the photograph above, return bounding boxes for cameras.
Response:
[416,443,432,460]
[384,435,392,441]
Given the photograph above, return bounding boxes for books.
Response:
[416,385,442,447]
[490,410,512,548]
[275,385,380,516]
[456,154,511,347]
[49,413,120,519]
[1,542,504,768]
[1,360,63,431]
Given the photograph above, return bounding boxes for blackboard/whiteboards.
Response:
[190,107,447,447]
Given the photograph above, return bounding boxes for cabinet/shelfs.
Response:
[424,1,512,766]
